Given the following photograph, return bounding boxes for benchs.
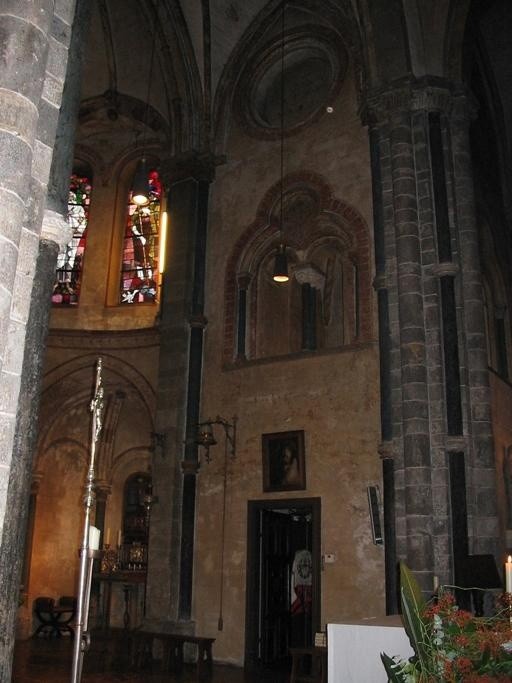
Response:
[103,623,219,676]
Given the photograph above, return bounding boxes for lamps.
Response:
[128,1,161,208]
[269,0,293,286]
[457,554,502,618]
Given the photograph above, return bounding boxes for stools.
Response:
[290,645,326,679]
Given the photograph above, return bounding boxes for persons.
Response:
[94,386,104,442]
[51,190,87,293]
[269,438,300,486]
[129,204,158,296]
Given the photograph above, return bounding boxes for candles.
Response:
[104,528,111,550]
[116,528,123,549]
[504,554,512,595]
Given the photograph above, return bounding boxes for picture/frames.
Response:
[260,428,309,494]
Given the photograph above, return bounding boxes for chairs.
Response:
[30,596,76,640]
